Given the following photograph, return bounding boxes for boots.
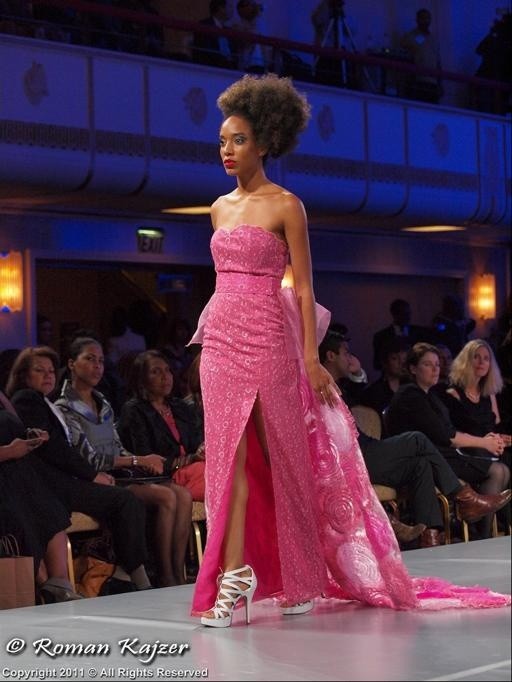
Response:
[386,511,426,541]
[451,484,512,524]
[418,528,439,548]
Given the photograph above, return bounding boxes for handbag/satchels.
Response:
[107,468,169,484]
[1,556,35,609]
[457,447,499,461]
[74,556,115,598]
[172,462,206,502]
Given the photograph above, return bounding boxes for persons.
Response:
[0,288,512,605]
[185,65,357,633]
[226,0,276,74]
[190,0,245,75]
[397,7,441,75]
[310,0,352,88]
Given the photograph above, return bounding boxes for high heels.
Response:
[41,577,84,604]
[201,564,258,628]
[280,592,326,614]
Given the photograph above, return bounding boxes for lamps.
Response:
[0,249,25,313]
[467,271,497,320]
[281,264,295,297]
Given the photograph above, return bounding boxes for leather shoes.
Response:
[103,576,138,595]
